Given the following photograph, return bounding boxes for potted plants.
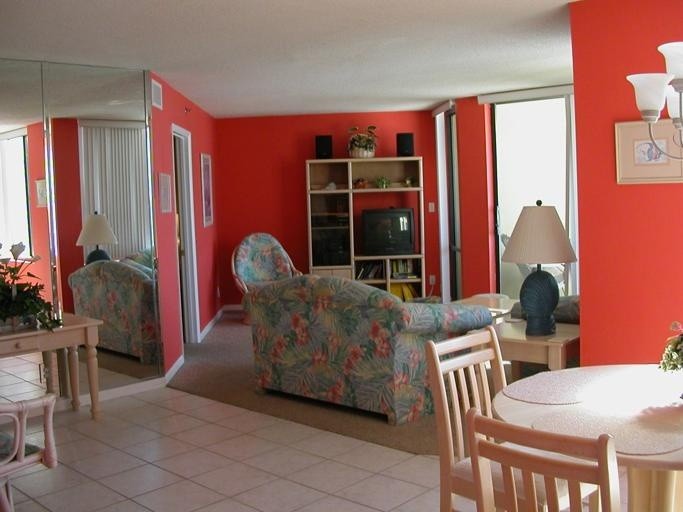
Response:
[347,125,379,157]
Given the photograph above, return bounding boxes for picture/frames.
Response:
[34,179,47,208]
[613,120,683,185]
[159,174,172,213]
[200,153,214,229]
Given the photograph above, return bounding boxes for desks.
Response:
[0,313,104,420]
[495,321,579,381]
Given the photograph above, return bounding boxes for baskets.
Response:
[348,134,376,158]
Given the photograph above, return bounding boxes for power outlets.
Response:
[430,275,436,285]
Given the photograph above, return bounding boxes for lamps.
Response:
[626,41,683,160]
[76,211,119,260]
[501,200,577,337]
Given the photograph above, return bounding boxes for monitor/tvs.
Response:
[360,208,416,256]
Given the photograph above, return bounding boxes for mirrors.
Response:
[0,59,164,413]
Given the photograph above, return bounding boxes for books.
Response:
[356,259,419,302]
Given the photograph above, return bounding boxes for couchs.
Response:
[68,252,160,365]
[249,275,492,427]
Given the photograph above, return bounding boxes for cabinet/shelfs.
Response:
[305,156,425,303]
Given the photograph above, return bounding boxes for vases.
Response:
[0,285,64,329]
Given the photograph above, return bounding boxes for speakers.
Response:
[315,134,333,160]
[397,132,415,157]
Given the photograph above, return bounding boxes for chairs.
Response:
[230,232,304,325]
[424,326,507,512]
[0,394,57,512]
[466,407,621,512]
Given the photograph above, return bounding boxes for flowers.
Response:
[0,241,41,313]
